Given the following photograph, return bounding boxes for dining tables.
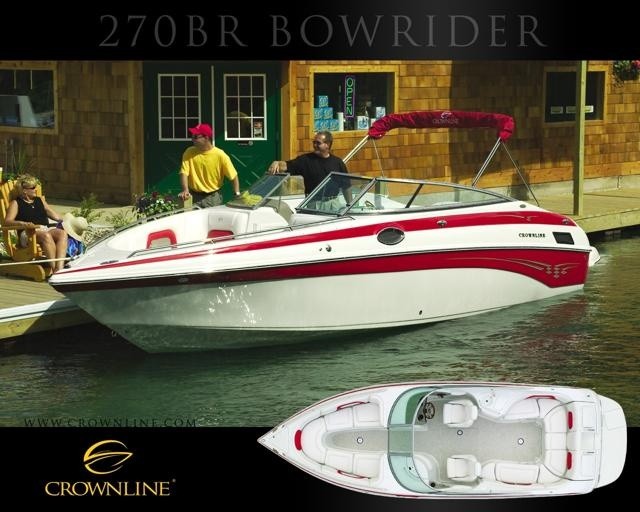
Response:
[612,60,640,81]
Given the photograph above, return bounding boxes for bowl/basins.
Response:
[28,203,35,208]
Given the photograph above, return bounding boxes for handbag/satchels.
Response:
[234,192,241,196]
[346,204,350,206]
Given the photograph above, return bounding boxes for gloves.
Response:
[48,111,591,353]
[255,381,627,499]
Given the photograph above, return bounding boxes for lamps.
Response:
[0,176,83,282]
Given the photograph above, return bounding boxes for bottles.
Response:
[61,212,89,242]
[188,123,212,137]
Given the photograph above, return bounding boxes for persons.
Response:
[268,131,353,212]
[4,175,67,273]
[254,118,264,138]
[178,124,241,209]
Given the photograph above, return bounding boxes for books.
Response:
[314,96,386,132]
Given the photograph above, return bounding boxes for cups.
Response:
[313,140,326,145]
[24,187,35,190]
